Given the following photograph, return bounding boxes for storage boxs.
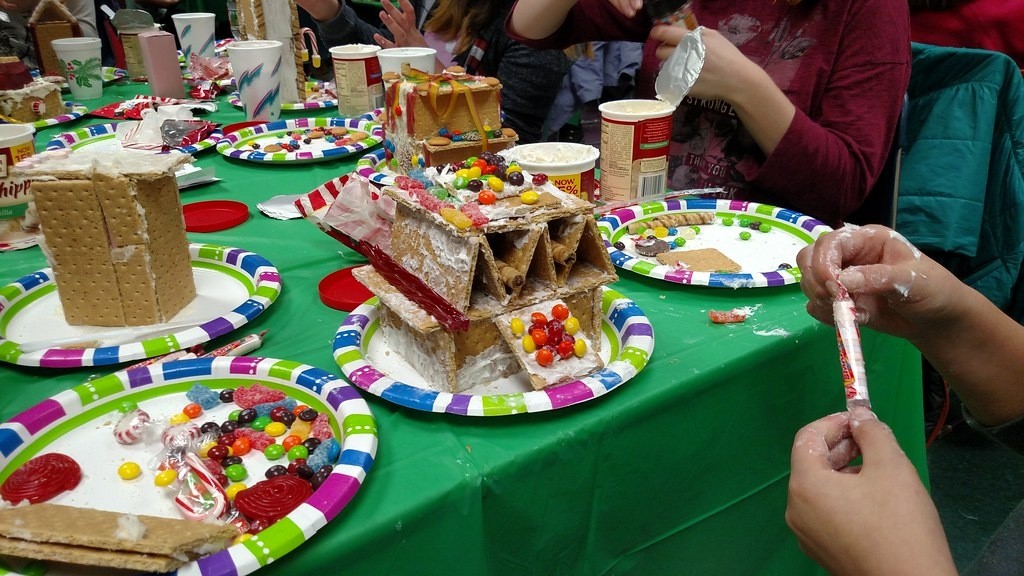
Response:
[138,29,186,99]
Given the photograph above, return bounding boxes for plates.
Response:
[332,285,654,416]
[46,123,223,155]
[0,242,281,368]
[216,117,383,164]
[29,67,127,92]
[0,101,89,127]
[227,82,338,109]
[360,108,506,124]
[0,356,378,576]
[596,198,835,288]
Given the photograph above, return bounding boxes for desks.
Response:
[0,70,932,576]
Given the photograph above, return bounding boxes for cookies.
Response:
[236,0,308,104]
[626,210,742,272]
[0,0,82,123]
[11,149,198,326]
[351,66,620,396]
[264,126,367,152]
[0,502,243,573]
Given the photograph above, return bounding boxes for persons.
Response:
[296,0,510,70]
[507,0,911,233]
[786,225,1024,576]
[0,0,98,72]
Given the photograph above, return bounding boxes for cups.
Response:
[508,141,600,204]
[0,124,42,219]
[376,47,437,92]
[119,23,162,83]
[328,44,384,115]
[598,100,676,204]
[226,40,283,121]
[51,38,102,100]
[171,13,216,72]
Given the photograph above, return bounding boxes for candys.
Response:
[247,126,349,151]
[1,384,341,537]
[708,308,745,323]
[614,217,792,270]
[830,269,871,413]
